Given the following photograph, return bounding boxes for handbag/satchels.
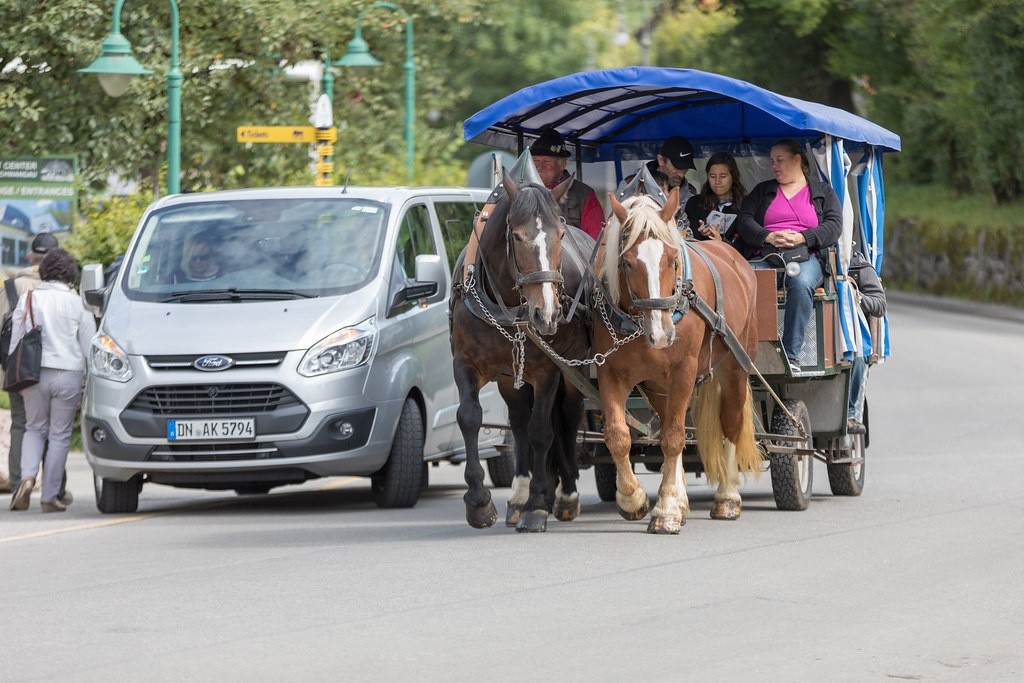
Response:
[2,290,42,391]
[761,241,809,267]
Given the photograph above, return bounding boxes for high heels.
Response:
[10,477,35,510]
[41,497,65,512]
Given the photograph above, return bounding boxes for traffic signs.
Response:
[237,124,316,144]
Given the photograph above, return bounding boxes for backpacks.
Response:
[0,279,18,369]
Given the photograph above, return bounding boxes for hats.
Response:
[32,233,58,253]
[660,135,697,171]
[529,129,572,158]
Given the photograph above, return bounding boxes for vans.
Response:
[78,184,518,515]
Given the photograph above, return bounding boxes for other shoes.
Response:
[59,490,73,504]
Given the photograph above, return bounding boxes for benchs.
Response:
[776,246,844,372]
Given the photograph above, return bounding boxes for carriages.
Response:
[449,66,901,534]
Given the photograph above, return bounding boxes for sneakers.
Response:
[788,357,801,372]
[847,419,866,433]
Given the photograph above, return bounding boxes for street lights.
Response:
[75,0,184,195]
[331,3,415,183]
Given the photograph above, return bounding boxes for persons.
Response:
[9,249,97,512]
[529,128,606,241]
[685,152,754,261]
[846,251,888,433]
[0,233,73,505]
[618,137,698,240]
[167,224,230,286]
[736,140,843,372]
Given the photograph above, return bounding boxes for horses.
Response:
[450,164,600,534]
[585,186,768,534]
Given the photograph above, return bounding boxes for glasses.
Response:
[190,254,208,261]
